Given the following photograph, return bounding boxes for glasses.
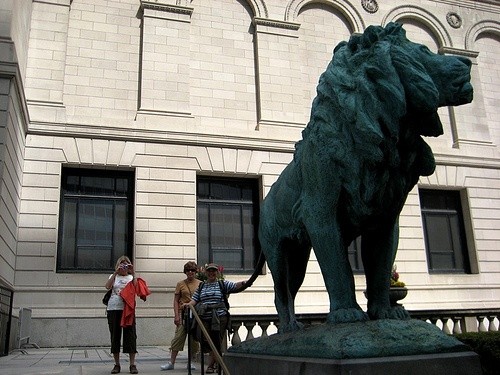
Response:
[186,268,196,272]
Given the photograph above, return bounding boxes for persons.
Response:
[183,263,253,373]
[105,256,138,374]
[161,262,205,370]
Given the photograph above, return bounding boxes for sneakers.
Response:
[160,362,174,370]
[190,362,196,369]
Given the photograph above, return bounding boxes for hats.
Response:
[205,263,218,271]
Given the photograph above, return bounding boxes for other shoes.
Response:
[111,364,121,374]
[130,365,138,374]
[206,367,214,373]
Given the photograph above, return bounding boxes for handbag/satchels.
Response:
[102,276,115,306]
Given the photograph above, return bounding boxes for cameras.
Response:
[122,265,128,269]
